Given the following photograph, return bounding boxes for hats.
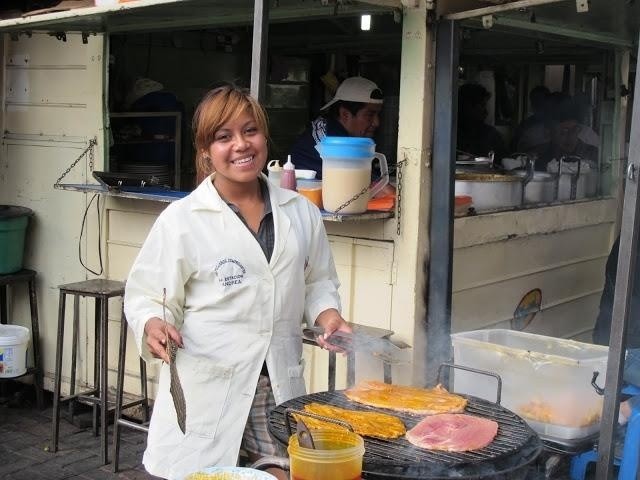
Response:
[319,77,385,111]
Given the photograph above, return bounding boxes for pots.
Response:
[454,151,605,212]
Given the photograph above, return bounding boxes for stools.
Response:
[302,319,394,393]
[1,270,45,412]
[50,278,151,474]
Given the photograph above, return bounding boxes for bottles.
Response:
[267,155,296,191]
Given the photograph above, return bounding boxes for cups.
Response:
[287,428,366,480]
[294,177,321,211]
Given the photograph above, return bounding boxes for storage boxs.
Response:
[451,327,630,441]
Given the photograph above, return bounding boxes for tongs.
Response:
[300,326,407,366]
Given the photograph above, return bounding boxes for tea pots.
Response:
[314,134,389,215]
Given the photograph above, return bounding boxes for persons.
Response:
[457,83,508,167]
[122,86,353,477]
[284,77,384,181]
[521,110,598,166]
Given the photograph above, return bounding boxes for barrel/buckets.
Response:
[0,324,30,378]
